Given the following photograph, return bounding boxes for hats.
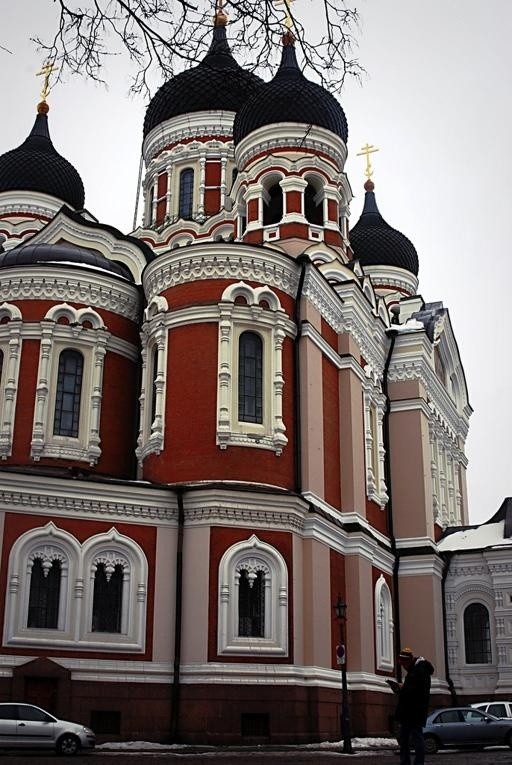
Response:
[398,646,413,661]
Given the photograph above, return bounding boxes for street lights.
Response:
[333,591,354,752]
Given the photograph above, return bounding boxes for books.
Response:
[384,678,402,687]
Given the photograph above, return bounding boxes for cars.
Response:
[461,701,512,721]
[0,703,96,757]
[420,706,512,753]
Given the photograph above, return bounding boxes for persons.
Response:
[387,646,435,765]
